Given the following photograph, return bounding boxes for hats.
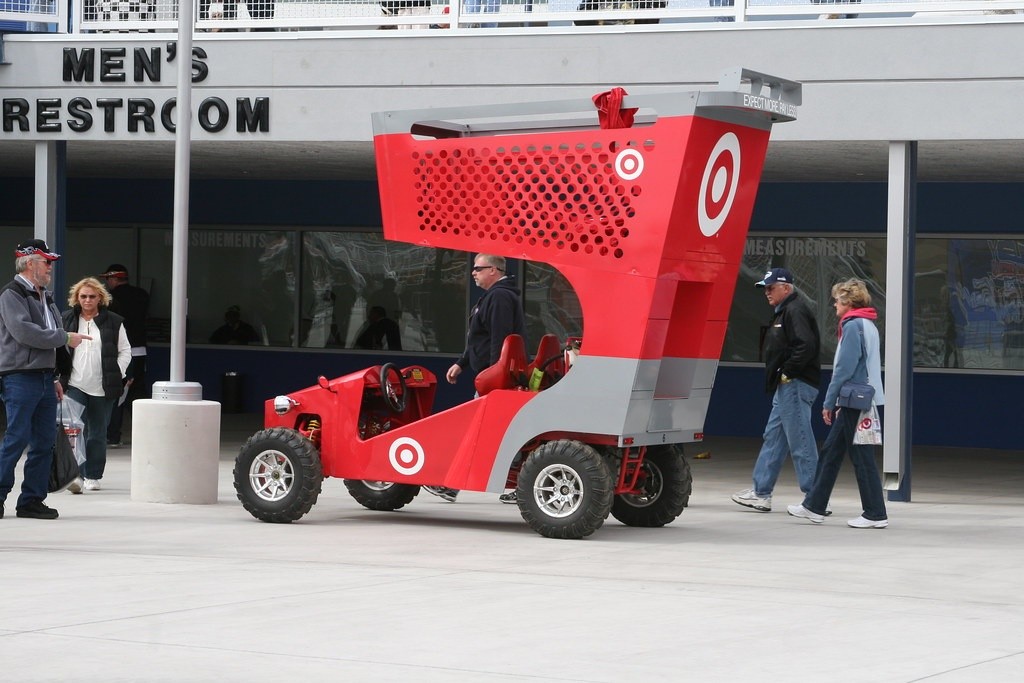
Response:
[16,239,61,261]
[754,268,794,288]
[97,264,129,278]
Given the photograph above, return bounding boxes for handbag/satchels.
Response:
[838,381,875,411]
[56,393,87,466]
[48,397,80,493]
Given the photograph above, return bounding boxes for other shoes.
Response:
[110,439,123,448]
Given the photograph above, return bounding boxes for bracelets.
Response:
[67,332,70,345]
[781,373,787,379]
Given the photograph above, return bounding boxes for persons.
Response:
[98,264,148,448]
[787,277,890,529]
[731,268,833,516]
[354,305,402,352]
[56,277,131,495]
[288,289,353,348]
[263,262,465,354]
[207,305,261,346]
[421,253,525,504]
[943,285,968,369]
[0,239,93,520]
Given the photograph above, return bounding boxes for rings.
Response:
[823,417,825,419]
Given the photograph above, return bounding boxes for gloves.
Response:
[775,361,798,381]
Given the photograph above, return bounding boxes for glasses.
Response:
[80,294,96,299]
[29,258,52,265]
[473,266,505,272]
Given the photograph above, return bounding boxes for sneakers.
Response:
[16,501,59,519]
[83,476,100,490]
[731,489,771,511]
[787,504,824,523]
[847,515,888,528]
[69,479,84,494]
[499,490,517,504]
[422,485,460,502]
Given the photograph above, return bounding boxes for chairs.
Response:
[527,335,564,383]
[475,335,527,396]
